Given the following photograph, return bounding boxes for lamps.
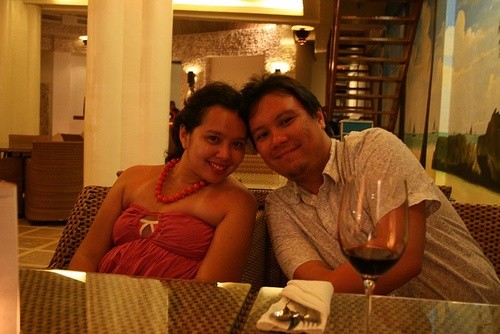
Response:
[79,35,88,45]
[292,25,314,45]
[183,63,203,95]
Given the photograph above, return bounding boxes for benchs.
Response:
[49,185,500,291]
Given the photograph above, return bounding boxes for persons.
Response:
[238,72,500,305]
[169,100,179,122]
[66,82,258,283]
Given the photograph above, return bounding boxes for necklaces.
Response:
[155,157,207,204]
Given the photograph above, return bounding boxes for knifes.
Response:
[288,305,304,330]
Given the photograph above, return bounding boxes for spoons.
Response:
[271,300,296,321]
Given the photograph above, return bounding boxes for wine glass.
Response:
[338,171,408,334]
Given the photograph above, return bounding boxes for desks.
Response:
[18,267,500,334]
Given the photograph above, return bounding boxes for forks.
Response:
[304,308,321,328]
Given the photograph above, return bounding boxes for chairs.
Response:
[0,133,84,225]
